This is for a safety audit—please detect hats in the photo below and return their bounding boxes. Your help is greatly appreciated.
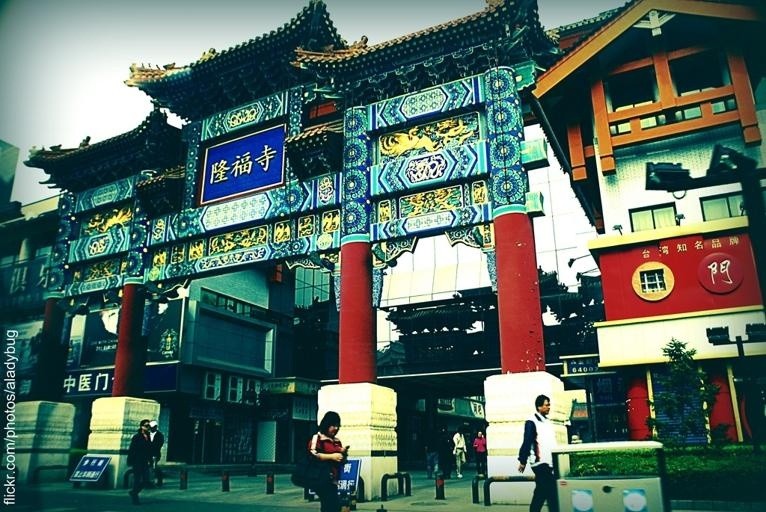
[149,421,158,428]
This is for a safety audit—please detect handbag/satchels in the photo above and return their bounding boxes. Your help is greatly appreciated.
[290,447,331,490]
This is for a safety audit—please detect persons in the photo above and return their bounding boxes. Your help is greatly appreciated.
[305,409,347,512]
[432,427,457,481]
[145,420,165,488]
[125,419,155,506]
[451,428,468,478]
[516,395,566,511]
[425,441,439,481]
[473,431,487,477]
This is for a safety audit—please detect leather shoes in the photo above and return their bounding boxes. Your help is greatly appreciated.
[129,490,141,505]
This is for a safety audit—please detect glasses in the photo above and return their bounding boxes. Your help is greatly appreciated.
[142,424,150,428]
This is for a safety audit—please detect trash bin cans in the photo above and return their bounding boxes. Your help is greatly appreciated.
[552,441,672,512]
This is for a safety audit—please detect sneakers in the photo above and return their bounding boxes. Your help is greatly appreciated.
[457,474,463,478]
